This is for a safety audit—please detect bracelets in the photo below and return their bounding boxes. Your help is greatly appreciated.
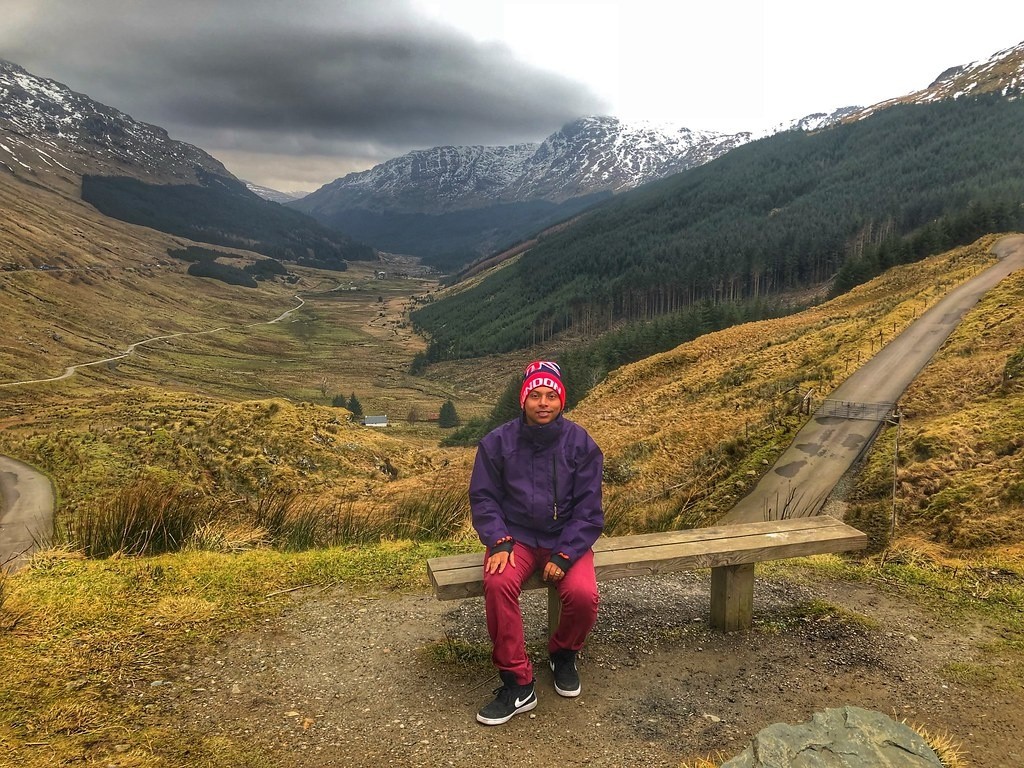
[558,552,569,559]
[494,536,513,549]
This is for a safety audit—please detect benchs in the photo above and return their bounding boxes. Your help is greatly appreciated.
[427,516,869,641]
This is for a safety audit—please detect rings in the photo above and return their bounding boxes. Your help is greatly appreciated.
[556,570,561,574]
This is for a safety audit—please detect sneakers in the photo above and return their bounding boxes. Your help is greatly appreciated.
[476,671,537,726]
[548,650,583,697]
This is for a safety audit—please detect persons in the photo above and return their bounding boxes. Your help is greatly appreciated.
[468,361,603,731]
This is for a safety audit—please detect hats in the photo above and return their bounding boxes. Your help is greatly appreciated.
[520,361,566,414]
